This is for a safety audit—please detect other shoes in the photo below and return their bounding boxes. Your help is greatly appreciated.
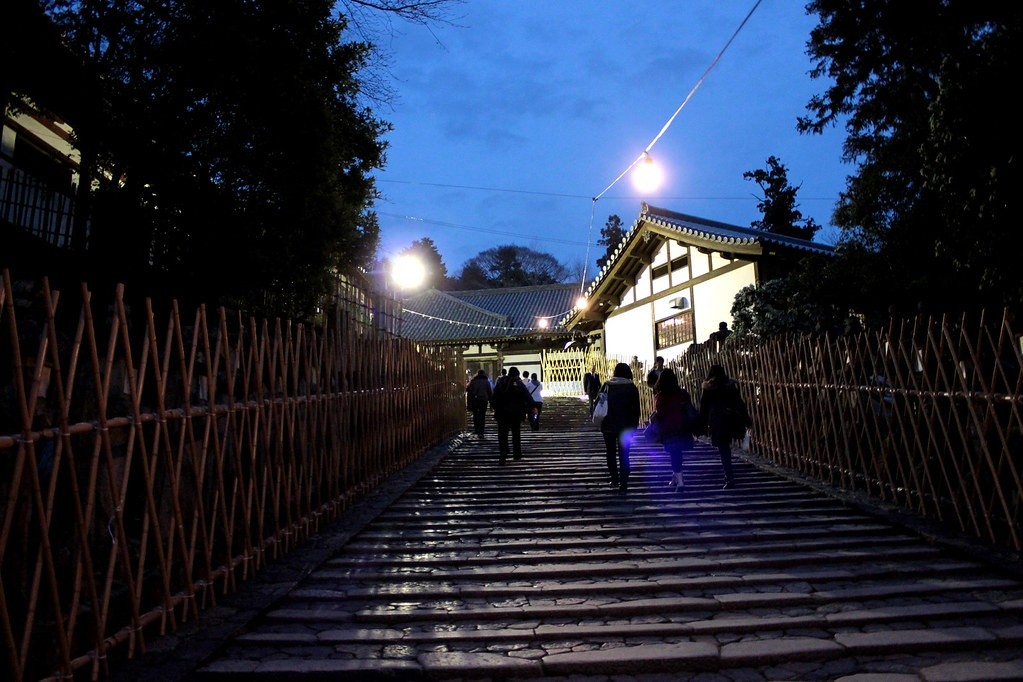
[499,450,507,466]
[609,482,619,488]
[477,433,484,439]
[723,475,735,490]
[513,453,521,461]
[618,489,626,495]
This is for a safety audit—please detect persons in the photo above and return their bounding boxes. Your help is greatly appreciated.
[644,368,699,493]
[700,365,753,490]
[710,322,733,344]
[584,365,601,417]
[485,369,507,413]
[522,371,543,431]
[466,370,494,439]
[592,363,641,495]
[491,367,538,464]
[629,356,643,381]
[647,357,665,412]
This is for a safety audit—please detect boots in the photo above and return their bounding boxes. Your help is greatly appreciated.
[669,472,685,492]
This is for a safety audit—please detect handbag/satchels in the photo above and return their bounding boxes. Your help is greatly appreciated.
[591,383,609,426]
[725,414,746,441]
